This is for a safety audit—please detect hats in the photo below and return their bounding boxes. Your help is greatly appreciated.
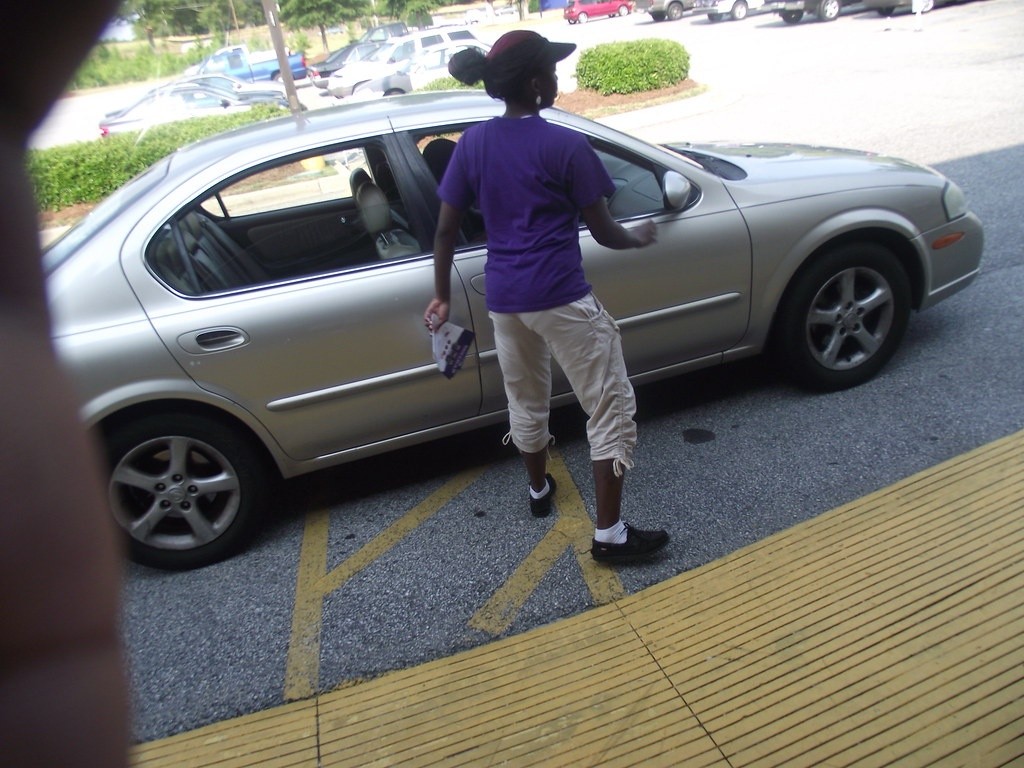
[482,30,577,84]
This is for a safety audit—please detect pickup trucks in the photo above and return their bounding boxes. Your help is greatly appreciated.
[215,45,291,62]
[196,51,307,83]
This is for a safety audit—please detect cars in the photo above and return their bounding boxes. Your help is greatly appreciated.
[352,39,492,96]
[647,0,965,23]
[99,72,290,137]
[358,22,409,39]
[564,0,633,24]
[308,40,383,98]
[31,95,987,575]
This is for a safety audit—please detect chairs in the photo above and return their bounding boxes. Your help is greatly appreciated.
[158,209,271,297]
[349,166,424,264]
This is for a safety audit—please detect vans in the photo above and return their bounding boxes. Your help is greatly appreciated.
[326,27,478,94]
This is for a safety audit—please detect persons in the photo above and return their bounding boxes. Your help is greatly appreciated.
[423,30,669,563]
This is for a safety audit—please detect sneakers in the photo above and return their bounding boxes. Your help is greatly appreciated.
[529,473,556,518]
[591,523,670,561]
